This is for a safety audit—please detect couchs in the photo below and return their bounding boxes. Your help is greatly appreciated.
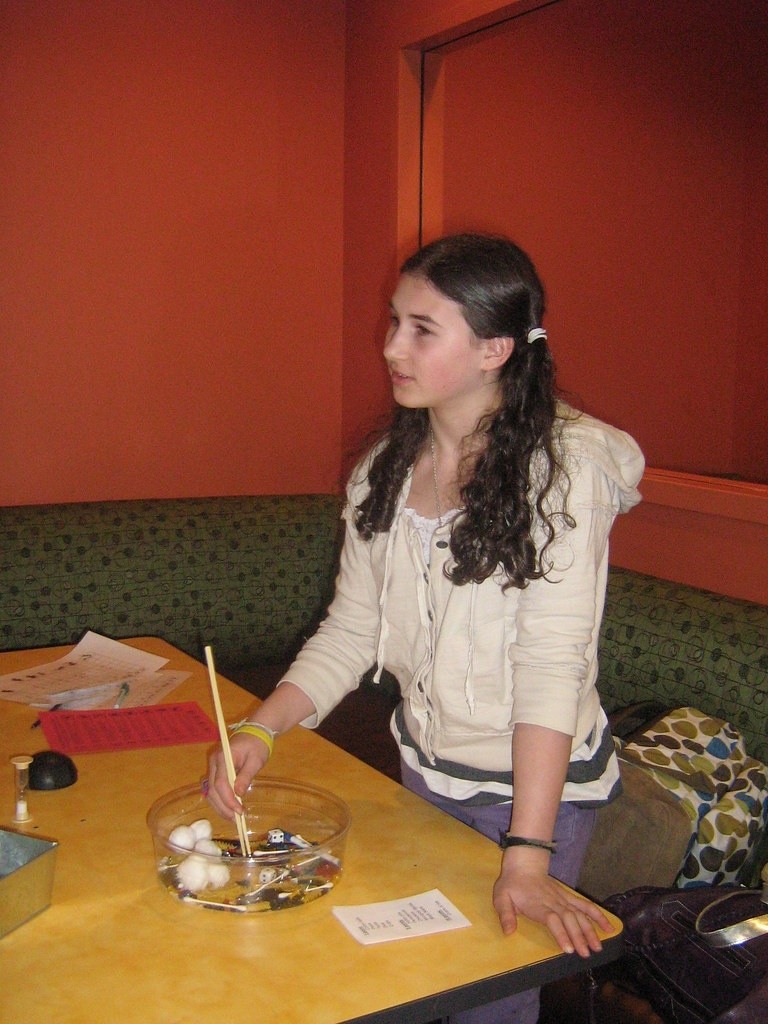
[0,495,768,1024]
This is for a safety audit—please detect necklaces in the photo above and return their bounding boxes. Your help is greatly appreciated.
[430,427,443,527]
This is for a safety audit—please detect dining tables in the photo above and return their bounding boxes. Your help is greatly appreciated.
[0,633,625,1024]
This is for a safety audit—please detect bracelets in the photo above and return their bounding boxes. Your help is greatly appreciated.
[502,836,556,852]
[227,717,274,757]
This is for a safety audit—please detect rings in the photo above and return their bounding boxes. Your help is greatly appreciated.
[202,778,210,797]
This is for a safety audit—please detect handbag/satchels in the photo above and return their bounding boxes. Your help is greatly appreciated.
[590,883,767,1024]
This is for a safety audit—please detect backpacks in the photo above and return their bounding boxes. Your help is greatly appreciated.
[575,698,768,950]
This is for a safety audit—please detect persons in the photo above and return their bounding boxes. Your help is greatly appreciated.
[204,230,645,1024]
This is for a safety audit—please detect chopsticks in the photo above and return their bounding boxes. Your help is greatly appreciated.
[205,645,252,857]
